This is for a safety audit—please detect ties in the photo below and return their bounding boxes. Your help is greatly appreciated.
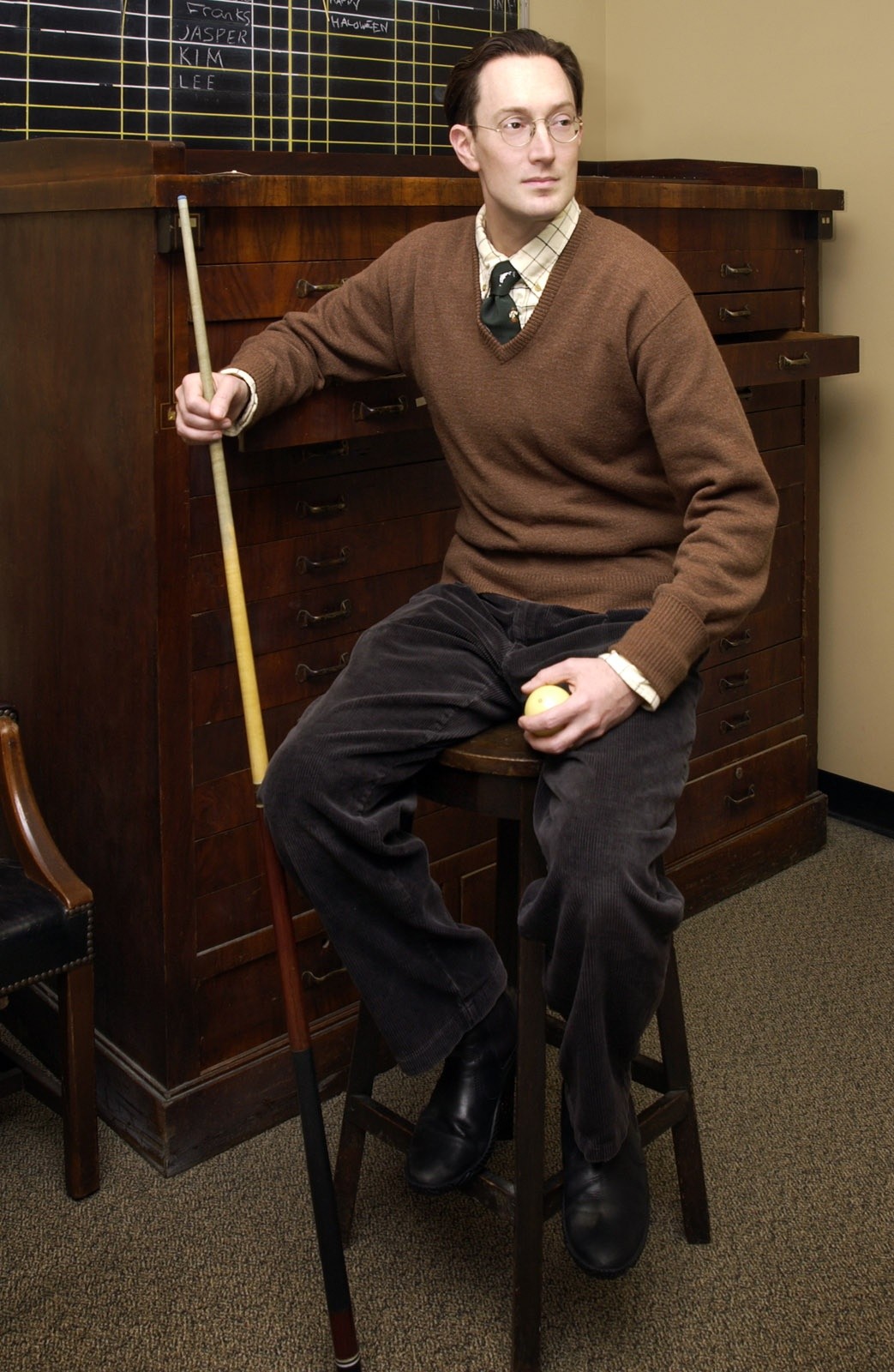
[479,259,522,347]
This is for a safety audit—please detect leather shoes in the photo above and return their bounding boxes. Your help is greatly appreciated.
[559,1085,653,1278]
[403,995,517,1196]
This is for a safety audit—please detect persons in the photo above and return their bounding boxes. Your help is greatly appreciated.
[173,28,777,1276]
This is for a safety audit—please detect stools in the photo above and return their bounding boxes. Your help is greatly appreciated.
[331,717,712,1372]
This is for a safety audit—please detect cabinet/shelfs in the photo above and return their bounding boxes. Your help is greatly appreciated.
[0,140,858,1175]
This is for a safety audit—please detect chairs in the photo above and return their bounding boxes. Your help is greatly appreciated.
[0,717,99,1199]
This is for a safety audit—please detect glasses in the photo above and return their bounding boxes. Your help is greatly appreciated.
[464,110,583,146]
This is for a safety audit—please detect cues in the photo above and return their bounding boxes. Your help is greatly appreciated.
[175,194,368,1372]
